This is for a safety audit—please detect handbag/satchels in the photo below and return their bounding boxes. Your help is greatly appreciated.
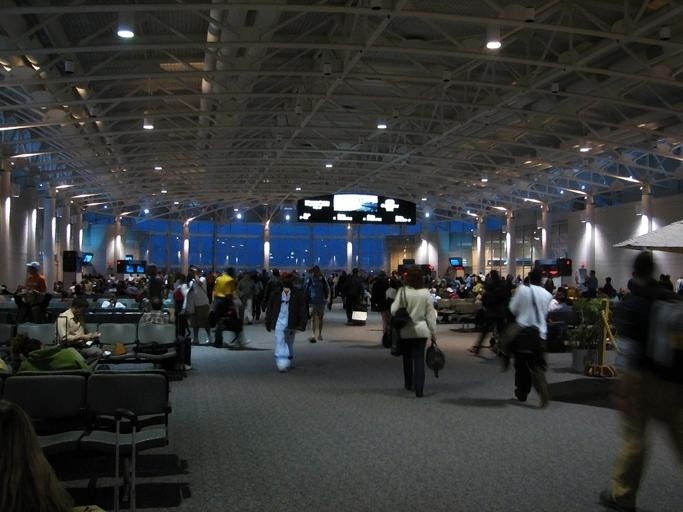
[426,340,446,374]
[391,306,413,330]
[514,326,541,358]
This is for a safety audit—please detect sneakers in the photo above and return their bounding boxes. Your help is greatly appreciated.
[467,339,497,357]
[598,487,640,512]
[401,384,424,398]
[192,316,323,373]
[517,395,549,408]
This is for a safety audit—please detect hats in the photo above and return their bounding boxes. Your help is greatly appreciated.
[26,262,41,270]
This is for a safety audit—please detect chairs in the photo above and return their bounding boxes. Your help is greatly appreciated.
[0,286,196,512]
[541,305,616,349]
[429,287,486,332]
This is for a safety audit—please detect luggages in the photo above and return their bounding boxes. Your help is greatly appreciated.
[351,299,368,326]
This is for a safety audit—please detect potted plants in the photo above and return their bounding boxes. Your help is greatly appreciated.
[570,296,610,374]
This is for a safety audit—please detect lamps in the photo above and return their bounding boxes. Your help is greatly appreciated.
[114,10,136,39]
[63,57,74,74]
[141,108,155,131]
[273,1,672,143]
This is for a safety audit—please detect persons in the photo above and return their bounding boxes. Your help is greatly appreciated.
[598,250,682,511]
[0,400,106,510]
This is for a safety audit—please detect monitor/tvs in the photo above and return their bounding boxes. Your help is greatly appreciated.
[124,264,136,274]
[449,257,462,267]
[125,255,133,260]
[82,253,94,264]
[136,264,145,273]
